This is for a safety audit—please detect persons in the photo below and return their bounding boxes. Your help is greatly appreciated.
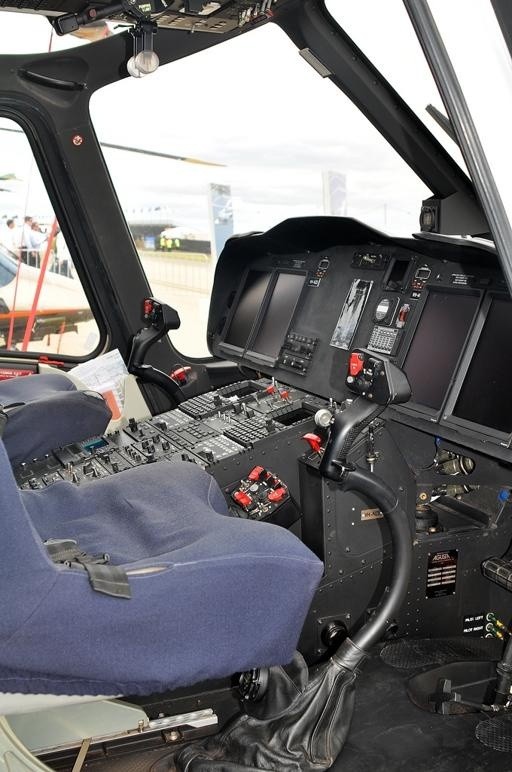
[5,218,17,251]
[29,222,51,268]
[16,216,38,266]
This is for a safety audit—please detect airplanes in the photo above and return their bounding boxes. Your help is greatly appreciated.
[0,242,95,347]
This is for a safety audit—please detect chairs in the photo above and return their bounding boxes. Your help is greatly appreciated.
[1,371,112,464]
[1,441,323,717]
